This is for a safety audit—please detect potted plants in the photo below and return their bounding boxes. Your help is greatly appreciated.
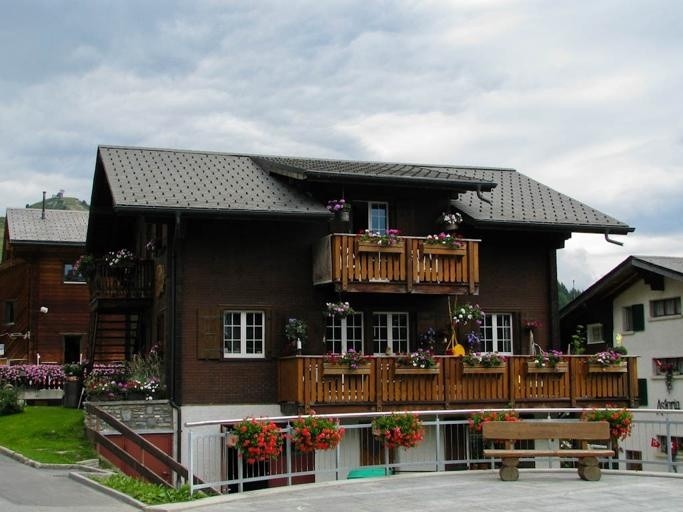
[275,312,311,353]
[63,362,83,382]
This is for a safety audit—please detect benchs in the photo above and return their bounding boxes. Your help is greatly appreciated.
[480,418,617,481]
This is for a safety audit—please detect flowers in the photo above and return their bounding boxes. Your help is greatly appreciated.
[324,198,350,213]
[339,347,366,369]
[529,348,564,369]
[370,406,425,452]
[359,227,465,251]
[86,359,163,403]
[436,210,463,226]
[226,407,348,465]
[0,362,65,385]
[404,299,508,370]
[468,406,522,445]
[580,403,635,442]
[591,349,624,372]
[323,301,357,318]
[523,318,545,334]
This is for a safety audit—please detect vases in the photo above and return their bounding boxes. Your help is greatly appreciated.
[338,211,349,221]
[445,224,459,232]
